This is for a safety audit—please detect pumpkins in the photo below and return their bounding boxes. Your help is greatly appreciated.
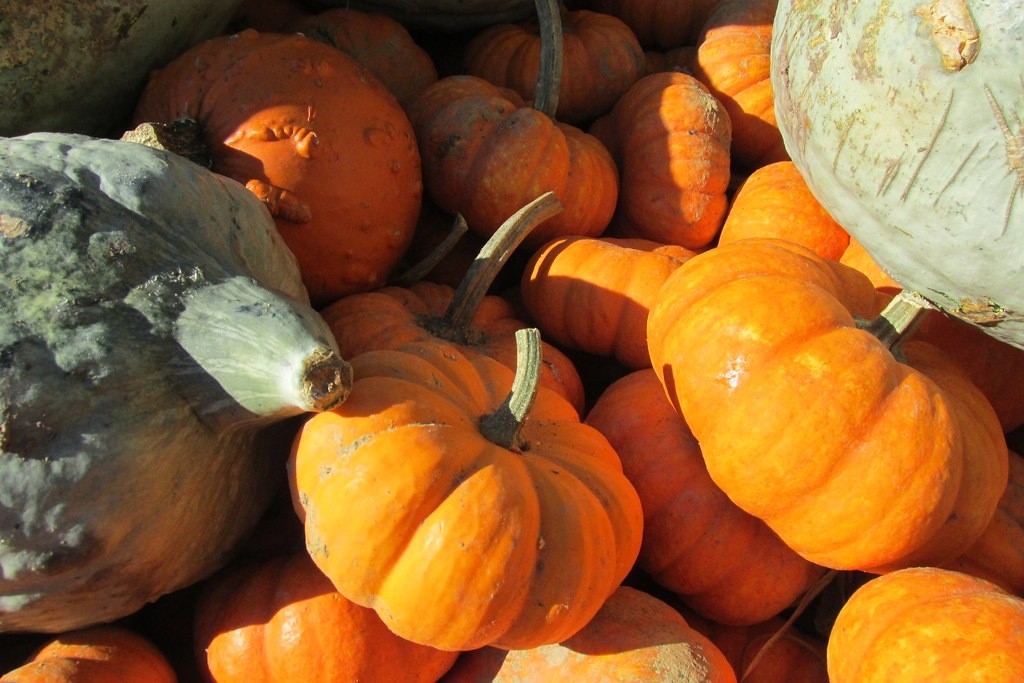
[0,0,1024,682]
